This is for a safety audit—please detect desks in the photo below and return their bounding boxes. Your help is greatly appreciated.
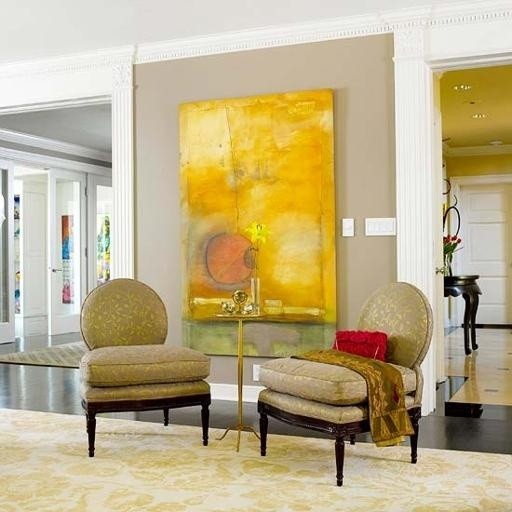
[442,274,482,355]
[214,312,266,453]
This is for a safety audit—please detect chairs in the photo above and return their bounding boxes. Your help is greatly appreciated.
[79,277,211,457]
[258,282,434,486]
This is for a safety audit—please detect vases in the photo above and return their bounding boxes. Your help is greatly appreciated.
[443,260,450,276]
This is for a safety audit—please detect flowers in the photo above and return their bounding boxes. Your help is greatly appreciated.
[442,234,464,274]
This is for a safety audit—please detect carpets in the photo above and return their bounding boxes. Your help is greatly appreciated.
[1,339,88,369]
[1,406,512,510]
